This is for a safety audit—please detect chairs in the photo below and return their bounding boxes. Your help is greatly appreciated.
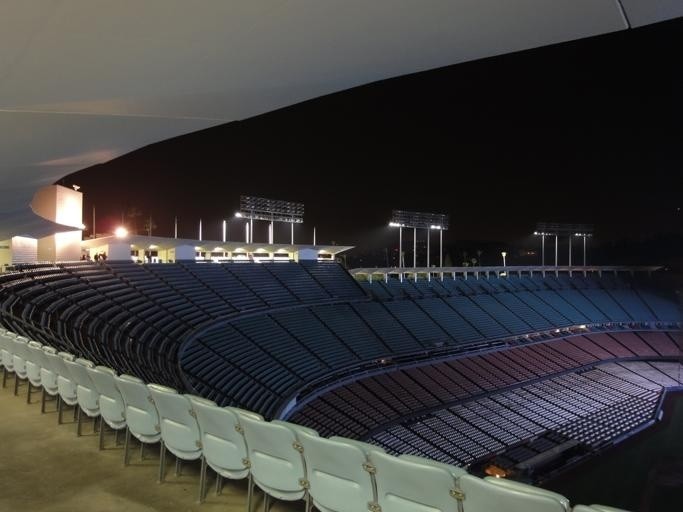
[0,327,631,512]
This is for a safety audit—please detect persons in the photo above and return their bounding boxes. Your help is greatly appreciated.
[82,252,108,265]
